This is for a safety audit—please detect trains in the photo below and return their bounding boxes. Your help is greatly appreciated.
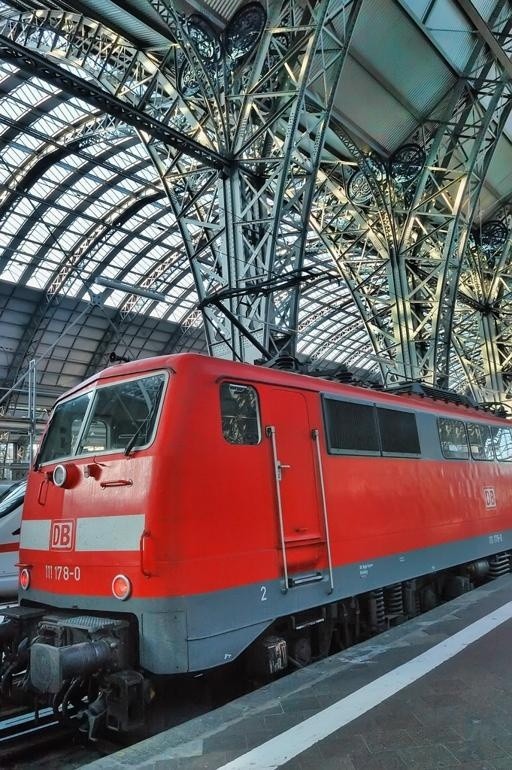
[1,472,33,604]
[0,350,512,754]
